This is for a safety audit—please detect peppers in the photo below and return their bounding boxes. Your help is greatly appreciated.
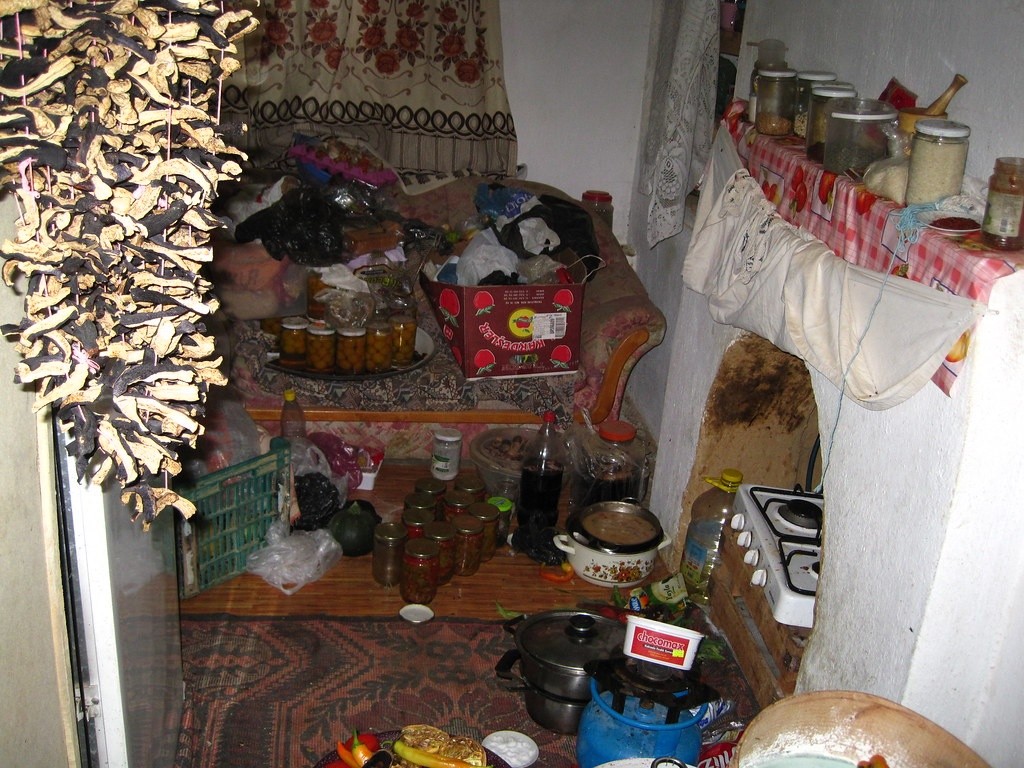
[378,740,494,768]
[539,560,574,583]
[495,600,527,618]
[324,728,401,768]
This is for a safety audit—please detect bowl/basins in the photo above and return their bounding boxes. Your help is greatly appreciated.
[622,616,705,670]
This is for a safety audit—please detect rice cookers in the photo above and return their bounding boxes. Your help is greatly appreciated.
[553,497,672,587]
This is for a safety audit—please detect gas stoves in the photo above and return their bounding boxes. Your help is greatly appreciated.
[731,483,824,628]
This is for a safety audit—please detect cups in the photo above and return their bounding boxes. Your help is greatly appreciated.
[758,40,784,70]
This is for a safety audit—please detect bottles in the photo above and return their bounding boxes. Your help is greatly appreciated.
[679,468,743,606]
[574,419,649,505]
[981,157,1024,251]
[280,270,417,374]
[582,189,613,233]
[373,475,518,606]
[511,410,565,552]
[431,428,462,480]
[750,61,970,207]
[280,389,307,438]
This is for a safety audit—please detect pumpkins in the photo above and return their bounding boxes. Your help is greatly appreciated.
[328,505,378,557]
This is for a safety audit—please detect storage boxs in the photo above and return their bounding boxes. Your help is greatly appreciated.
[418,243,588,381]
[175,436,294,597]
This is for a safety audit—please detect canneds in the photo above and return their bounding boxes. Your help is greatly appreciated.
[981,156,1024,251]
[431,428,462,480]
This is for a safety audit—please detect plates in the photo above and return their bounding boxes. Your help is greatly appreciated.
[312,731,512,768]
[916,210,983,236]
[265,326,435,381]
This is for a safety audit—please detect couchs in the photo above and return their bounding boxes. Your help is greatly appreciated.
[205,166,666,461]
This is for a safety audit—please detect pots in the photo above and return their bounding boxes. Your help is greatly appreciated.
[493,612,628,736]
